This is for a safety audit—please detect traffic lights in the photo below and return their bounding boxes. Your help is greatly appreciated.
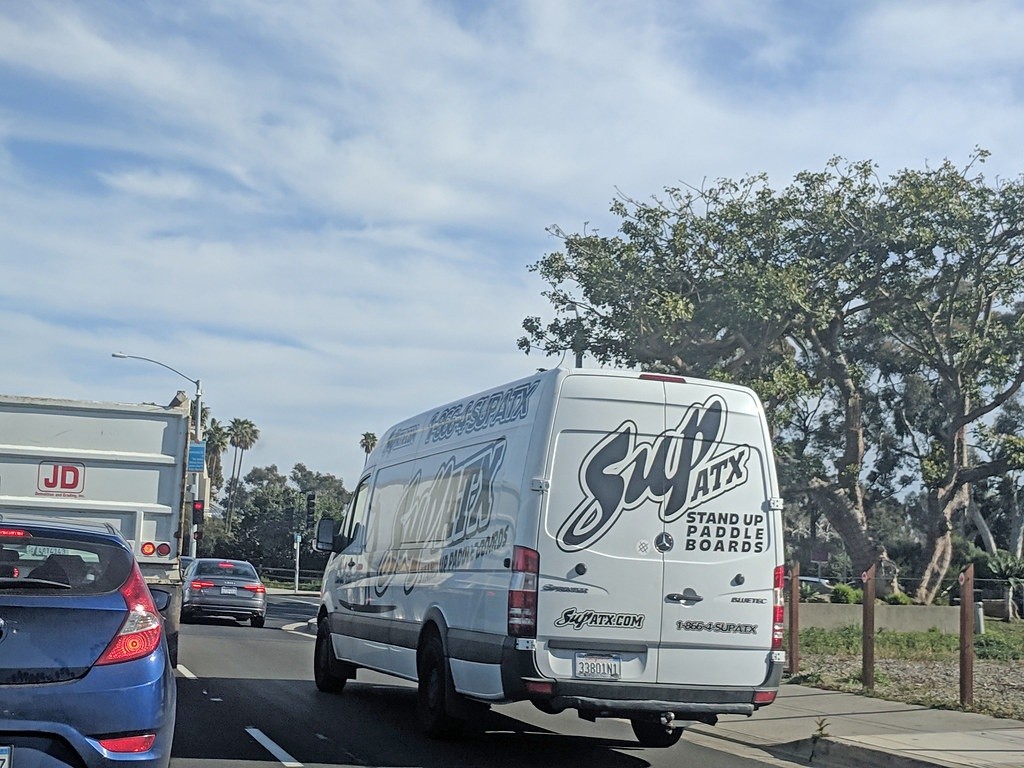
[191,499,204,525]
[305,494,315,528]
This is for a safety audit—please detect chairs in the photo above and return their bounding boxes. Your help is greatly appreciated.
[0,550,96,586]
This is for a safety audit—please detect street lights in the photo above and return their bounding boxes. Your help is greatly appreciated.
[110,351,206,559]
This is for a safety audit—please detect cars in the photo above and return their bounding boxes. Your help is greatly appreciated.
[0,515,177,768]
[175,554,193,581]
[783,574,829,604]
[180,559,267,628]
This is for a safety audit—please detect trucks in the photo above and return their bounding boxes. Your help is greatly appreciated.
[0,388,199,672]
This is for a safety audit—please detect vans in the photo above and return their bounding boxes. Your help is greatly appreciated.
[308,368,788,748]
